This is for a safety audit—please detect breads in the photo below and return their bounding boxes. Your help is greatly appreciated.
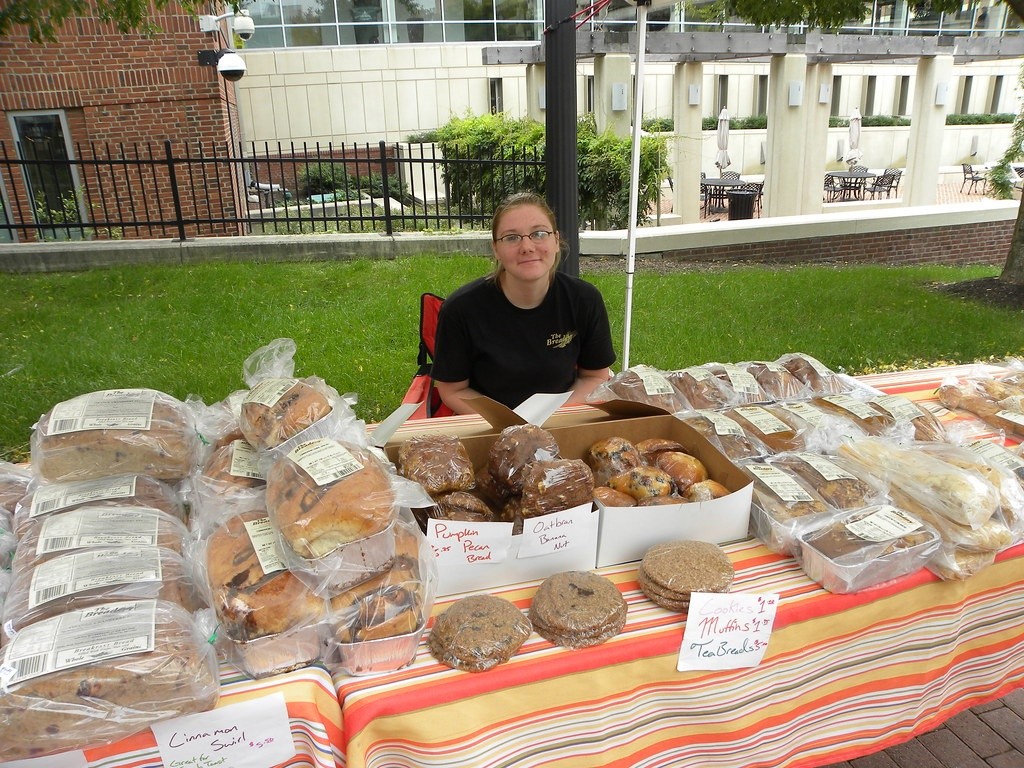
[399,423,735,534]
[0,380,423,766]
[608,355,1024,582]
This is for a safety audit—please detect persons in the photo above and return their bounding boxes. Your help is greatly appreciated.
[428,191,618,416]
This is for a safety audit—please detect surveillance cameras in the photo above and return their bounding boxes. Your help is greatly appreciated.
[218,53,247,81]
[235,17,255,40]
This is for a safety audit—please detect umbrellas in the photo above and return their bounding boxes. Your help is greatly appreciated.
[711,105,732,213]
[844,107,864,201]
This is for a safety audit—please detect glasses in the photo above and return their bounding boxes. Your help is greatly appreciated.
[496,230,556,246]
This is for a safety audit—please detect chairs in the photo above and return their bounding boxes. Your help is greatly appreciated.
[960,163,986,196]
[403,291,455,420]
[668,171,765,219]
[824,165,903,202]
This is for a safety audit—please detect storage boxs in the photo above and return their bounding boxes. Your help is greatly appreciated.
[372,395,601,599]
[545,399,757,568]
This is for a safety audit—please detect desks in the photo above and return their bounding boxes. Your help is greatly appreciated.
[829,172,877,201]
[701,178,746,219]
[0,362,1024,768]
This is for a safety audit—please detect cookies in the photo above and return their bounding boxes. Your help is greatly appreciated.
[525,571,628,649]
[637,542,734,615]
[426,595,532,673]
